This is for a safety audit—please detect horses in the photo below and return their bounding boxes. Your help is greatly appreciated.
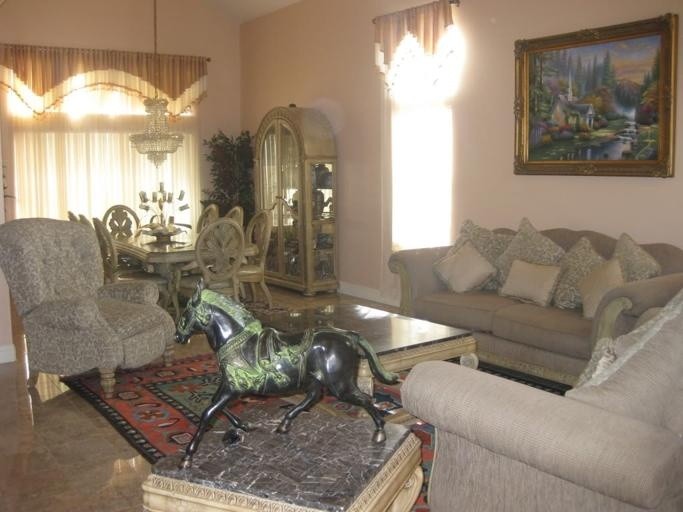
[171,276,403,471]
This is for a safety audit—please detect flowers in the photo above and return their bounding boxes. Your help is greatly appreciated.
[139,183,190,224]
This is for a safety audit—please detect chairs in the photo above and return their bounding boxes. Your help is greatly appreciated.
[0,218,176,399]
[400,307,683,512]
[68,203,273,321]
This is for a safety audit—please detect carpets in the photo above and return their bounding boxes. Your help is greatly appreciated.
[58,353,573,512]
[167,294,292,321]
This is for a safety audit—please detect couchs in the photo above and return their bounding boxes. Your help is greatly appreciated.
[388,228,683,386]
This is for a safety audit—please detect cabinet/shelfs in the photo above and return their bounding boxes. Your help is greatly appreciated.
[255,106,339,296]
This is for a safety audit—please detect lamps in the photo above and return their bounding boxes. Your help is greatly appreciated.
[129,0,184,168]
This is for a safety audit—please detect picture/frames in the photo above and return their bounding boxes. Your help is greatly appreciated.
[514,13,679,177]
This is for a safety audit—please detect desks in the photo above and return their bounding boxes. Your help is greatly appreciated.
[141,403,424,512]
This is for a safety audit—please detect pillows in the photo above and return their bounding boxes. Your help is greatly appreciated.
[577,289,682,387]
[433,217,660,319]
